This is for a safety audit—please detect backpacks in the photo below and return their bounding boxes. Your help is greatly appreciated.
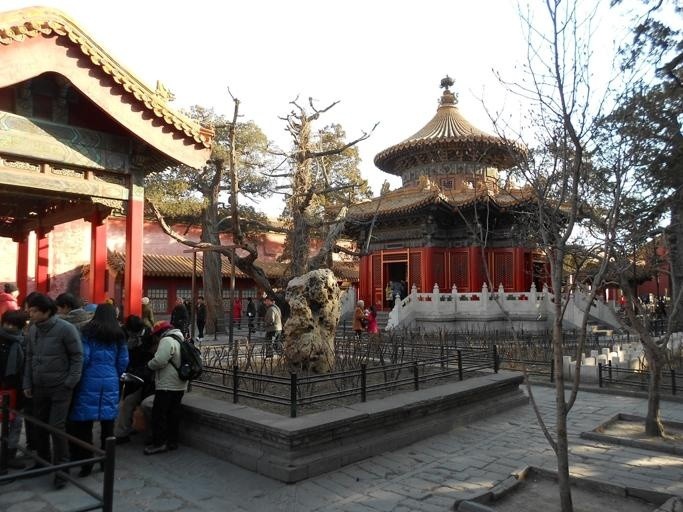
[162,326,203,383]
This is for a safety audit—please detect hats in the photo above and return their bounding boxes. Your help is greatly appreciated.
[147,319,174,336]
[125,315,144,332]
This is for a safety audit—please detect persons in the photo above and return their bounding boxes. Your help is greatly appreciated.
[352,300,367,341]
[385,279,406,309]
[232,292,285,357]
[365,306,377,334]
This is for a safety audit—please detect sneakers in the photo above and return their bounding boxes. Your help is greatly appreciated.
[142,439,178,456]
[16,449,37,458]
[75,465,93,479]
[51,464,72,490]
[116,436,130,444]
[6,461,25,469]
[24,460,54,479]
[129,428,137,436]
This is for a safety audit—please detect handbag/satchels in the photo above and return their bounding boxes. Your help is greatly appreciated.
[361,318,369,329]
